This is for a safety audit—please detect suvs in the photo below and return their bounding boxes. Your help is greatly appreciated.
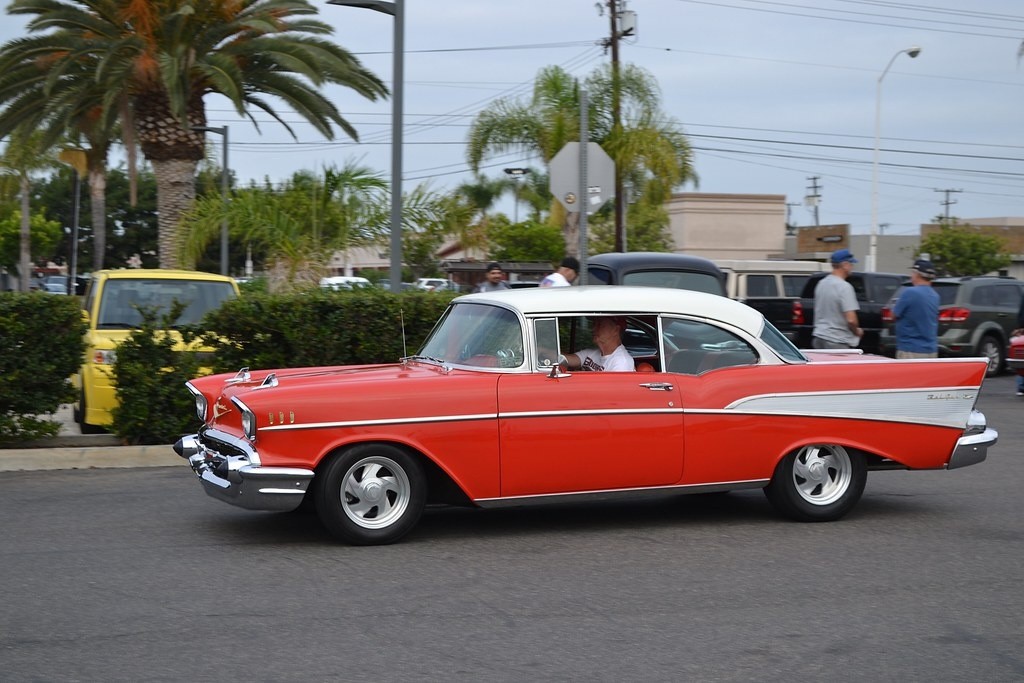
[876,275,1024,377]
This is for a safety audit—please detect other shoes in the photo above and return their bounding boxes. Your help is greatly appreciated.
[1016,388,1024,395]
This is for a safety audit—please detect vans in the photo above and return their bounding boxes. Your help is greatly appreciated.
[792,270,914,350]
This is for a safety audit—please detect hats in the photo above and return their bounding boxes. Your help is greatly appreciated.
[560,256,580,273]
[831,249,858,264]
[486,263,501,271]
[908,259,936,275]
[586,316,628,330]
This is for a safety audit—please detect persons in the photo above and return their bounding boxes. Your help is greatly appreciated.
[810,248,864,349]
[893,260,941,358]
[474,261,511,292]
[1017,376,1023,394]
[539,257,581,286]
[527,315,636,371]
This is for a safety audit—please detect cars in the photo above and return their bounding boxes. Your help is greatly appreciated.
[1003,333,1024,379]
[312,250,835,344]
[60,268,251,435]
[175,282,996,542]
[8,269,94,298]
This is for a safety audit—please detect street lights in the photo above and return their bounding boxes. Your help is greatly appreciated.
[868,44,924,270]
[325,0,408,292]
